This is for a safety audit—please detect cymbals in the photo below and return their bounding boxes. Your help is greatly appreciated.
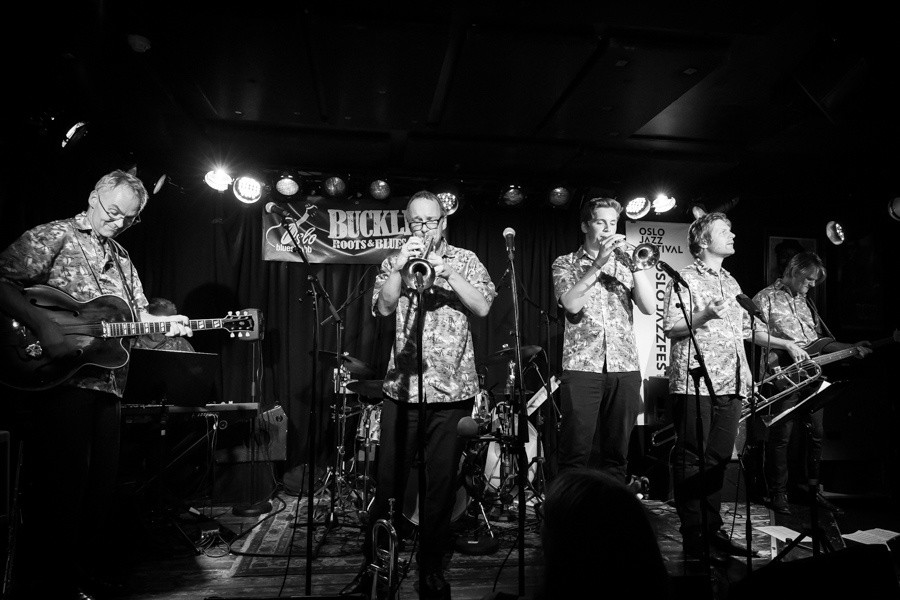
[345,380,384,399]
[309,348,379,375]
[492,387,535,396]
[488,344,543,361]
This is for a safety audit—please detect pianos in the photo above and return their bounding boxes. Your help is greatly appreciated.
[117,400,260,555]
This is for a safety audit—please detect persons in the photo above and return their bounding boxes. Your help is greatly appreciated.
[339,189,497,600]
[0,170,197,600]
[552,198,656,488]
[751,248,872,516]
[663,212,755,557]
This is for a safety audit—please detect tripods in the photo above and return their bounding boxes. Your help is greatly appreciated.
[760,378,852,562]
[286,360,364,522]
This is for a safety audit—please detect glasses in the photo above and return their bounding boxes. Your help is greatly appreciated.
[408,214,445,233]
[98,195,140,226]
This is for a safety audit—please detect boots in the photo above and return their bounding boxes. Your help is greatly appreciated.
[773,463,800,514]
[804,454,837,511]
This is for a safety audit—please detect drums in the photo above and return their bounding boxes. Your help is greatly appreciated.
[401,451,476,528]
[354,404,382,446]
[470,388,491,427]
[479,417,545,500]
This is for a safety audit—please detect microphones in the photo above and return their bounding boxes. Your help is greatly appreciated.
[265,202,293,218]
[736,293,769,325]
[660,262,689,289]
[376,266,381,275]
[495,270,510,293]
[520,354,536,375]
[502,227,517,260]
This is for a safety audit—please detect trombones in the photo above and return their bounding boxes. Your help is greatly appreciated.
[650,356,823,447]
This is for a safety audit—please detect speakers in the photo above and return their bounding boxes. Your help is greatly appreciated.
[231,406,289,462]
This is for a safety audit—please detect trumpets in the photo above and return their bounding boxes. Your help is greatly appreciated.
[602,236,660,270]
[367,497,398,599]
[400,236,436,291]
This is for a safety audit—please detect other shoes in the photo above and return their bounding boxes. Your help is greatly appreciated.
[341,571,371,595]
[683,536,734,558]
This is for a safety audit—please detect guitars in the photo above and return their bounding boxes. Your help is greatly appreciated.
[0,284,256,394]
[763,328,900,400]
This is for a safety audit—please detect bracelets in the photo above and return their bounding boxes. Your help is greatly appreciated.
[592,261,601,269]
